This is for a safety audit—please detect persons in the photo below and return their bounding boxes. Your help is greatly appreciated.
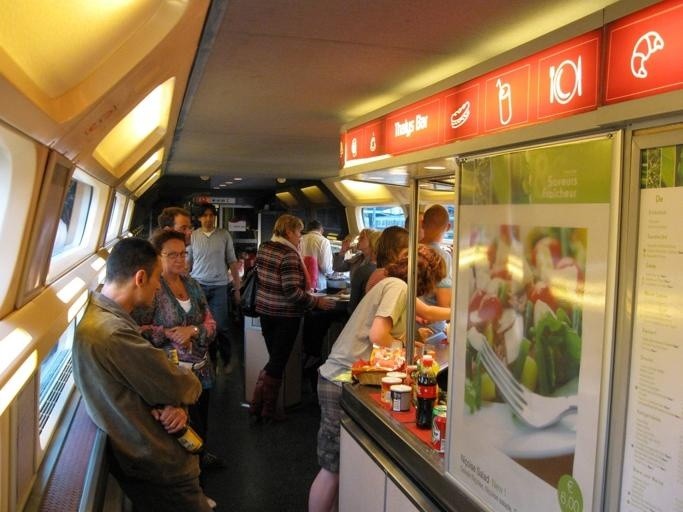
[72,237,214,512]
[307,243,451,512]
[132,203,241,487]
[297,204,481,392]
[248,213,337,422]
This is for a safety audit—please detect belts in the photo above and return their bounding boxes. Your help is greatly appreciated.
[179,358,208,370]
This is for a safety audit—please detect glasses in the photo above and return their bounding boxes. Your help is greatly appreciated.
[162,250,188,258]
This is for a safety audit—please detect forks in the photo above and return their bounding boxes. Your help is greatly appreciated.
[478,336,577,430]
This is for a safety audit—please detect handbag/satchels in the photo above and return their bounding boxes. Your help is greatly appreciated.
[240,271,259,317]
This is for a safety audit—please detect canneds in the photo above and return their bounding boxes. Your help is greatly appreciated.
[380,371,411,412]
[431,404,447,454]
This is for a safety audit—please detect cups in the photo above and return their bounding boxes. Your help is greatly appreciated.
[343,241,358,248]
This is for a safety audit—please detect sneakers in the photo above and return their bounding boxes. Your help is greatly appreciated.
[216,362,232,376]
[206,496,218,510]
[205,452,226,468]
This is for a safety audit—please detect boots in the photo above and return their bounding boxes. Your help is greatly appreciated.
[248,368,282,417]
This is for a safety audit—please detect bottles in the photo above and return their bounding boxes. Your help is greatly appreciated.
[155,406,206,455]
[169,349,179,366]
[415,357,437,429]
